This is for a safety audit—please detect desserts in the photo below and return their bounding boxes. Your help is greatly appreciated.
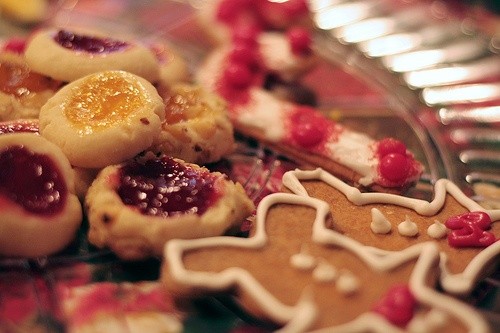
[0,25,500,333]
